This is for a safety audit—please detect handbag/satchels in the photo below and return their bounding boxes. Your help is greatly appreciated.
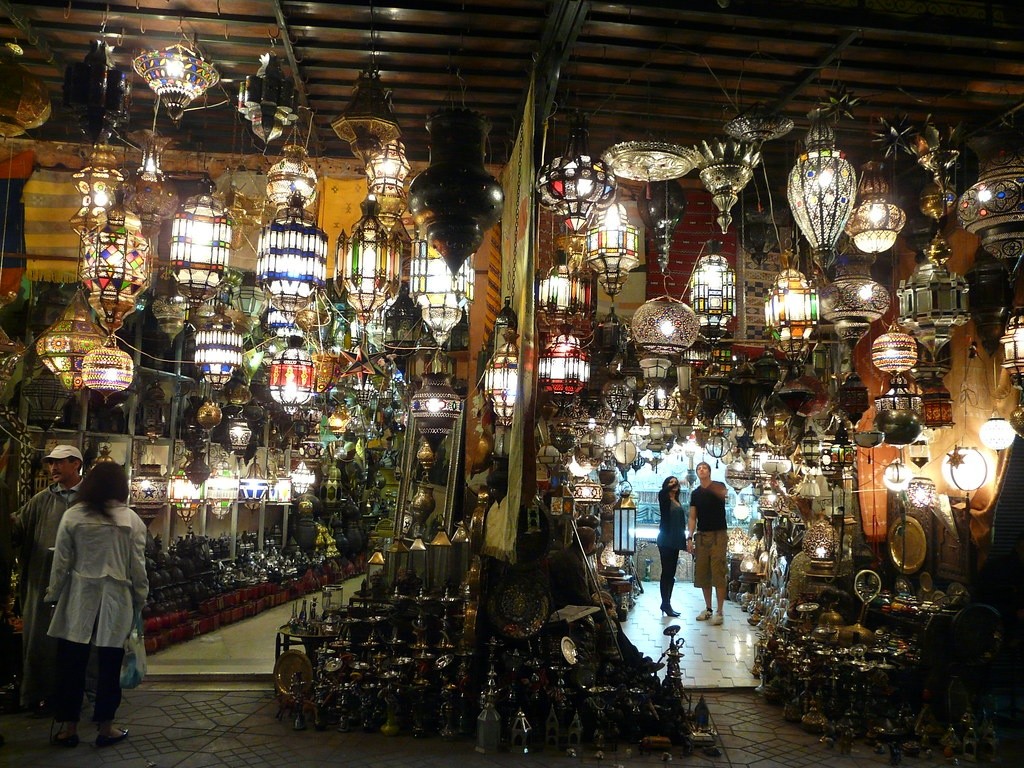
[118,607,146,688]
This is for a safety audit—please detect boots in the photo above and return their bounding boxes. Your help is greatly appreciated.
[660,577,681,617]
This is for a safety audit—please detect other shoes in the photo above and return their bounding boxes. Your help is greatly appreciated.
[696,608,712,620]
[713,615,723,625]
[53,729,79,747]
[96,726,129,746]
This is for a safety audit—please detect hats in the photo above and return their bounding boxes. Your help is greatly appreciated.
[43,445,83,462]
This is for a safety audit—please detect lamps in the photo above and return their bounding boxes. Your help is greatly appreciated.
[1,1,1024,613]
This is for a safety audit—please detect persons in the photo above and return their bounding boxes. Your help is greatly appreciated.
[657,475,687,617]
[678,462,730,626]
[0,444,86,717]
[43,461,150,749]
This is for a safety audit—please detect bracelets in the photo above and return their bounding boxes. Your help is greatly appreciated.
[687,537,692,541]
